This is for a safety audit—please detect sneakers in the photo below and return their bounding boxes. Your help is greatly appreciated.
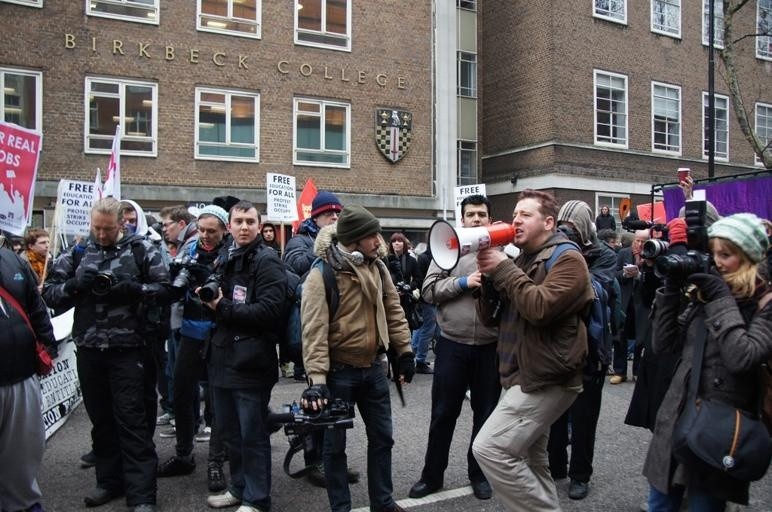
[85,487,124,506]
[207,465,263,512]
[610,375,626,384]
[157,456,195,476]
[80,451,96,466]
[306,464,358,487]
[156,413,211,442]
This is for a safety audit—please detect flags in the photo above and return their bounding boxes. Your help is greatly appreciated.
[104,124,122,202]
[92,167,103,207]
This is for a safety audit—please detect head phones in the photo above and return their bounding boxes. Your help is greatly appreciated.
[336,241,363,266]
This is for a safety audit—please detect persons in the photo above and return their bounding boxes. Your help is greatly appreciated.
[1,174,770,511]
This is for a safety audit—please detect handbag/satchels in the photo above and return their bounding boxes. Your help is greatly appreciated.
[410,303,424,330]
[672,397,772,481]
[35,341,53,375]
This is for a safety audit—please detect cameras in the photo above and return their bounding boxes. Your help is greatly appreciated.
[641,239,669,258]
[653,200,712,279]
[197,274,230,301]
[88,269,116,296]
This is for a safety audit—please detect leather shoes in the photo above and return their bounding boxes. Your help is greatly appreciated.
[471,480,492,499]
[569,480,587,499]
[409,482,442,498]
[416,362,434,374]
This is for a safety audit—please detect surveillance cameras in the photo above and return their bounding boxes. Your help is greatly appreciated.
[509,175,517,184]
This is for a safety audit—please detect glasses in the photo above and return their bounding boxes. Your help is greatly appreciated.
[322,211,341,216]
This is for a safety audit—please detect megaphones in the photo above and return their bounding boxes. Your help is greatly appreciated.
[429,214,515,273]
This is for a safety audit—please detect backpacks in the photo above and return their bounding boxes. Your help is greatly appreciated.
[251,246,339,362]
[547,243,612,374]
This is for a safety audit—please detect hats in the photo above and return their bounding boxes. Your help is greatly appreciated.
[311,190,380,246]
[707,212,772,263]
[198,205,229,226]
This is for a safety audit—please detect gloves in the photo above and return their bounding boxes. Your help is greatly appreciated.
[109,273,138,298]
[688,266,730,301]
[65,268,97,295]
